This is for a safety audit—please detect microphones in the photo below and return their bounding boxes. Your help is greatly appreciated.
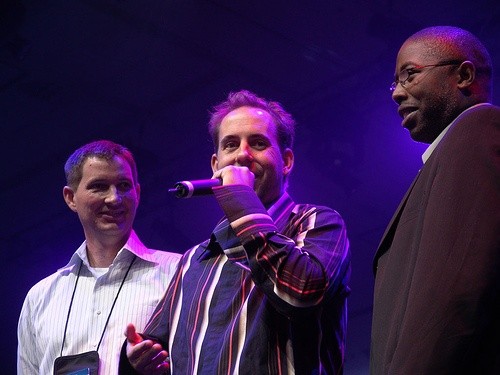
[168,178,222,198]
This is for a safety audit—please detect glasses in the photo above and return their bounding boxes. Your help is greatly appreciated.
[391,62,461,90]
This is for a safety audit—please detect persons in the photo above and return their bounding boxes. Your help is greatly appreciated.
[16,141,182,375]
[118,91,350,375]
[371,26,500,375]
